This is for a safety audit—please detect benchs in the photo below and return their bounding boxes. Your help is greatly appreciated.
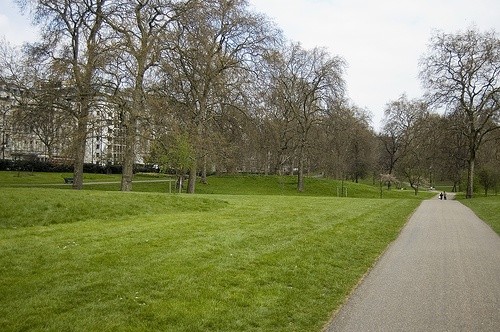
[64,178,73,184]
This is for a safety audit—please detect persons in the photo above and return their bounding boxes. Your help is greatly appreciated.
[439,192,444,200]
[442,190,447,200]
